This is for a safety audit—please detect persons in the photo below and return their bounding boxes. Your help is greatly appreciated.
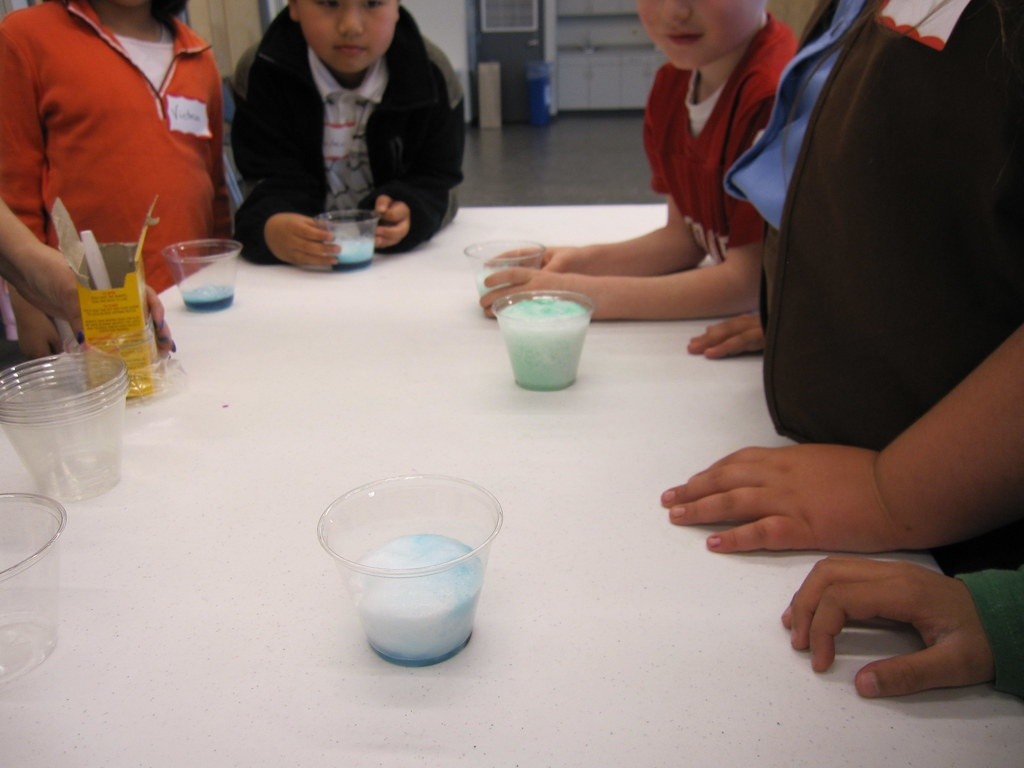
[231,0,466,276]
[0,0,236,363]
[478,0,1024,702]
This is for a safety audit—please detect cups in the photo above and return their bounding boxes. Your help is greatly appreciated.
[0,352,131,685]
[162,238,244,312]
[318,474,505,668]
[492,288,597,391]
[315,209,381,271]
[463,237,547,317]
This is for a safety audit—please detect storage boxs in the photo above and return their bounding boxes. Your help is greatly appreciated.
[50,194,165,398]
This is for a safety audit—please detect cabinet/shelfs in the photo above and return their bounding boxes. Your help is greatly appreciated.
[542,0,666,124]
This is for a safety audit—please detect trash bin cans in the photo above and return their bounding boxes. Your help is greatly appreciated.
[524,61,552,126]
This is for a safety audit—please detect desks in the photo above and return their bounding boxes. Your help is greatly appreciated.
[0,205,1024,768]
